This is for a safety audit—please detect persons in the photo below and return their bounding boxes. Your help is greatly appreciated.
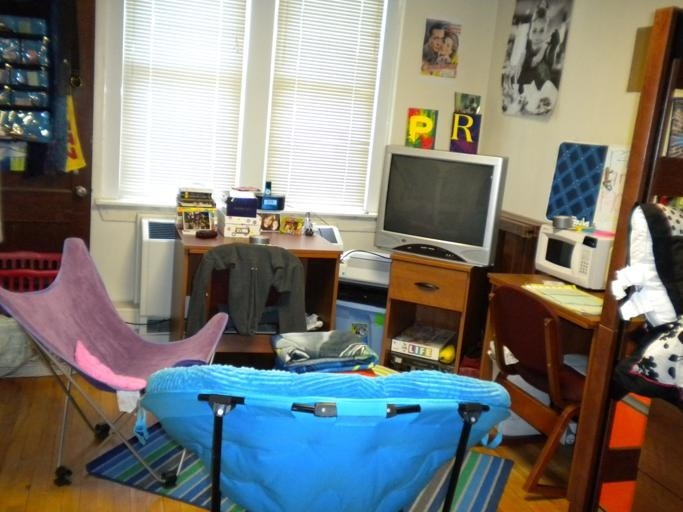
[500,0,573,115]
[436,32,459,65]
[422,21,448,65]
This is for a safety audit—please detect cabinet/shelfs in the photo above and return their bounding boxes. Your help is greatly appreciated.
[565,5,681,511]
[380,249,493,374]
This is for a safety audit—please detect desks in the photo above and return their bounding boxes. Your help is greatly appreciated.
[165,228,341,354]
[472,267,608,389]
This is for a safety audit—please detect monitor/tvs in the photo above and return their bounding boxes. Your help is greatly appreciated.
[374,144,510,268]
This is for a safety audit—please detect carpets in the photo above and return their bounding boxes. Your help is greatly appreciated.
[73,414,511,512]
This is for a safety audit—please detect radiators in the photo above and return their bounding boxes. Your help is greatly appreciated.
[134,215,192,322]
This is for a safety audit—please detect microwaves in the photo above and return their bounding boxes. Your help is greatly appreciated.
[533,223,615,291]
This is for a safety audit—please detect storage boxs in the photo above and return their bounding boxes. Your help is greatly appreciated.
[220,192,257,218]
[216,208,261,239]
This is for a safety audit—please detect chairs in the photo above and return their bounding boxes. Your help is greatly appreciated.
[477,280,586,490]
[126,364,509,512]
[191,243,308,355]
[0,231,229,492]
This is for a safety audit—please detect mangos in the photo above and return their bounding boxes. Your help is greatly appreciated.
[440,344,455,363]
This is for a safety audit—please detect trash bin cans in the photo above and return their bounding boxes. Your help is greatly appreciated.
[488,340,550,437]
